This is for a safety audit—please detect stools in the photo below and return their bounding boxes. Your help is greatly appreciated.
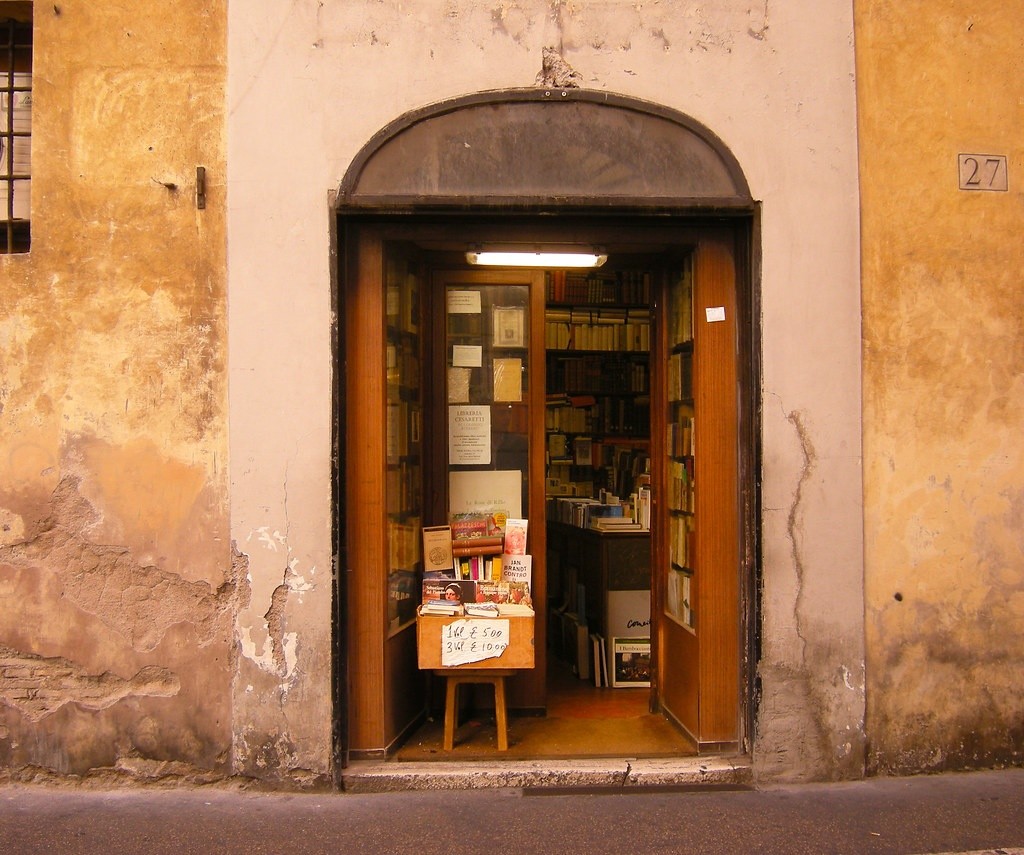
[433,671,518,751]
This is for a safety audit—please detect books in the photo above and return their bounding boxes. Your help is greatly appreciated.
[666,271,695,630]
[447,267,652,532]
[386,254,425,633]
[547,589,650,688]
[420,515,535,618]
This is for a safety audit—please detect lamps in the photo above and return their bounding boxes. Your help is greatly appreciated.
[466,253,609,268]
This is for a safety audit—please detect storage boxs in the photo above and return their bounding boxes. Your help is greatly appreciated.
[415,603,535,670]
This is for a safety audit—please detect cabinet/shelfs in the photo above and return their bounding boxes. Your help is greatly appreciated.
[546,302,653,690]
